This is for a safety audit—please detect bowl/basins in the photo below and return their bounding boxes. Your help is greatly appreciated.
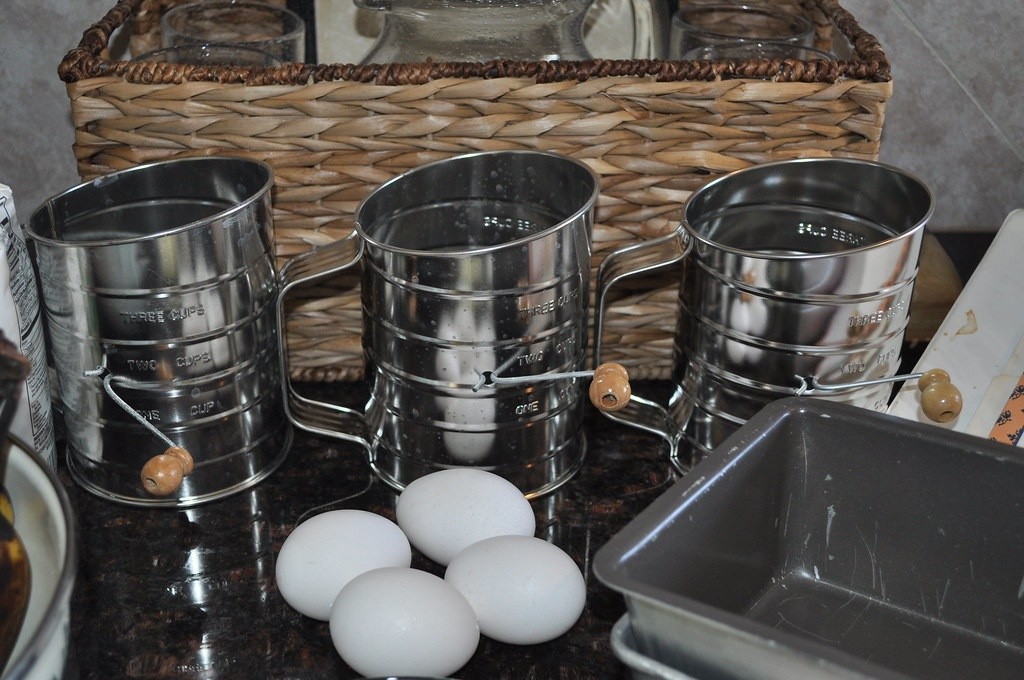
[0,430,81,680]
[588,391,1023,680]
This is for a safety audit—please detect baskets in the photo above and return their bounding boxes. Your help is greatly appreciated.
[57,1,890,382]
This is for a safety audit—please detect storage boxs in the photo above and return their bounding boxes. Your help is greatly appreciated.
[593,398,1024,680]
[58,1,895,380]
[272,149,599,504]
[599,158,935,489]
[24,156,288,510]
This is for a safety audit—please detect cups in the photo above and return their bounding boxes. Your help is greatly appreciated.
[26,148,294,507]
[693,41,837,63]
[128,42,283,77]
[587,153,962,486]
[267,148,634,503]
[159,0,306,69]
[665,7,817,64]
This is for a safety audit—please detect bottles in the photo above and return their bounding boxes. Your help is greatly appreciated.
[348,0,653,62]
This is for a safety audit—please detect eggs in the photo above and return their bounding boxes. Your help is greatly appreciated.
[276,467,587,680]
